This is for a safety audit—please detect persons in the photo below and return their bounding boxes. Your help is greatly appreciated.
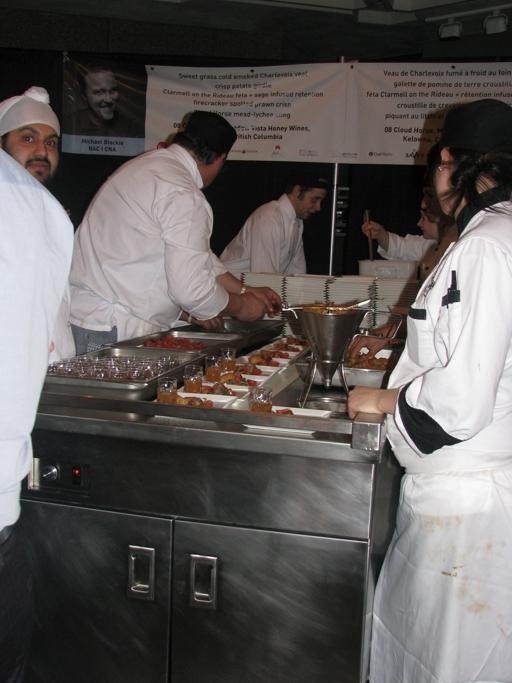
[64,60,145,136]
[70,109,285,350]
[0,79,64,185]
[345,98,511,682]
[218,170,330,276]
[179,309,228,330]
[0,146,79,683]
[358,186,454,261]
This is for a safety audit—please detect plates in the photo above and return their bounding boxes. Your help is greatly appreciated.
[241,268,421,334]
[148,337,394,439]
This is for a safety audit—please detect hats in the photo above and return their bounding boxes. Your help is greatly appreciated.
[185,110,237,154]
[427,98,512,164]
[0,85,61,137]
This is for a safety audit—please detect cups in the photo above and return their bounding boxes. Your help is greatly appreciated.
[48,354,178,379]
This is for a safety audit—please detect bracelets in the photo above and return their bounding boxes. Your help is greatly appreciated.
[188,314,193,322]
[240,285,249,294]
